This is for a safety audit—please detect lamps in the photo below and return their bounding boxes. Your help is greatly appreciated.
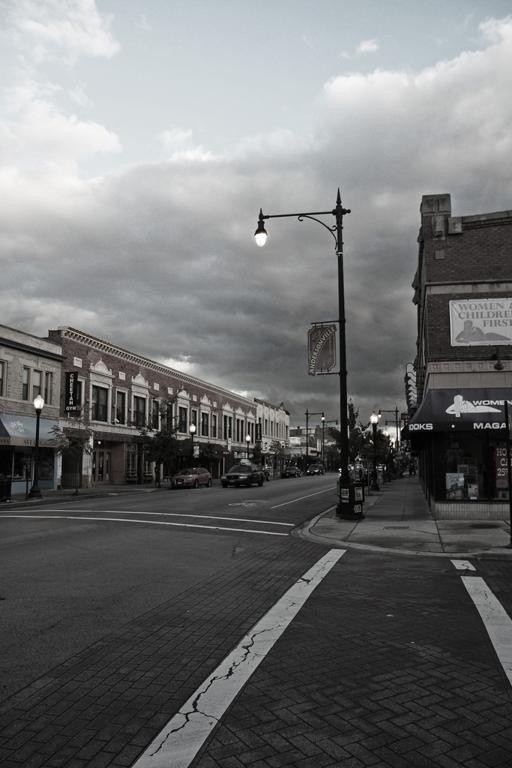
[491,352,505,370]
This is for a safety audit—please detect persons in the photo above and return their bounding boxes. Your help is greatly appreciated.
[359,468,362,478]
[154,463,160,488]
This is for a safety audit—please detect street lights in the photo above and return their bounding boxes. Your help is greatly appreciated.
[246,434,252,458]
[253,187,355,516]
[321,416,326,465]
[189,423,197,468]
[369,406,402,491]
[29,393,45,497]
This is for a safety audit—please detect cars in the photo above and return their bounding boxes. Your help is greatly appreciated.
[222,459,327,489]
[171,468,213,489]
